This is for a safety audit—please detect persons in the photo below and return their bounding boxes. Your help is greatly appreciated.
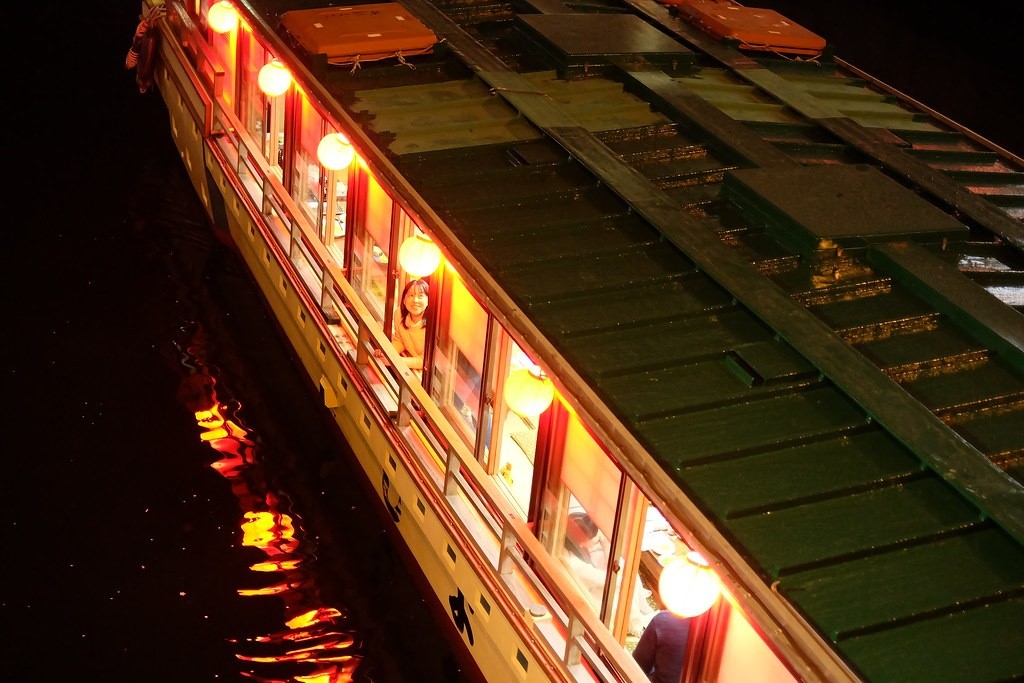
[632,609,691,683]
[373,279,429,370]
